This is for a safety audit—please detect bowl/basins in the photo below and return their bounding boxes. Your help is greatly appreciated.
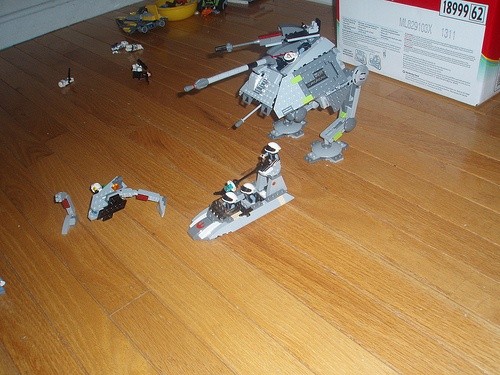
[153,0,198,21]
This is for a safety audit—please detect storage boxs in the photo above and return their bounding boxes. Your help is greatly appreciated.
[335,0,500,107]
